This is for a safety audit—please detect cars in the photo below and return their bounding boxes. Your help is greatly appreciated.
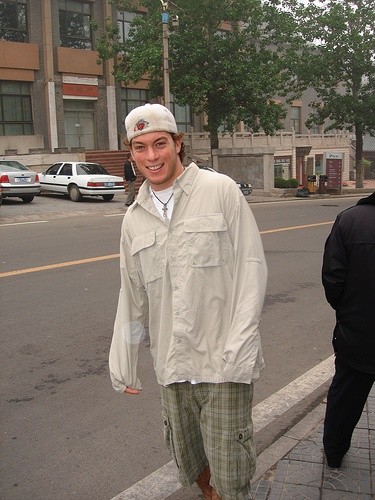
[198,165,252,196]
[0,160,42,203]
[32,161,125,202]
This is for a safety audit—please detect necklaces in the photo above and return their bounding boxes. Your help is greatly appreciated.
[152,190,174,218]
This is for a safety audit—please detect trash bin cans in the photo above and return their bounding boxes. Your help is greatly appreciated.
[319,175,328,194]
[307,175,316,193]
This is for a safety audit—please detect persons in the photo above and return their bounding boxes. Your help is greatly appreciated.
[124,153,137,206]
[322,192,375,468]
[109,104,267,500]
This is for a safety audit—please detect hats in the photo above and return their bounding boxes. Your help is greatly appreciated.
[124,102,178,145]
